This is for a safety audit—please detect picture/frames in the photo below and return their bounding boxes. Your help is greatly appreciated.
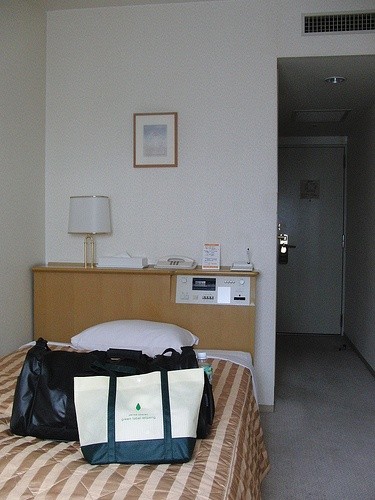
[133,112,178,167]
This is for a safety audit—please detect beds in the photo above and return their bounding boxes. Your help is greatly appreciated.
[0,340,271,499]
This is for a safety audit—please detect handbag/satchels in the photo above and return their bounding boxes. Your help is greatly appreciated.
[9,337,215,442]
[73,368,204,465]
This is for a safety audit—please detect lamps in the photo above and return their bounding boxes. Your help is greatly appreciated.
[68,196,112,268]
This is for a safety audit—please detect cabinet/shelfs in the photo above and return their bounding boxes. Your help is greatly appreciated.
[32,265,259,364]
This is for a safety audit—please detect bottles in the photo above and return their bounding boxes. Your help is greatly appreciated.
[197,352,213,384]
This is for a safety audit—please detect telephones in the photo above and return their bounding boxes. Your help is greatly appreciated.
[153,255,198,269]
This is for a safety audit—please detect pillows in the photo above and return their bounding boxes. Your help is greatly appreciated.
[71,319,199,357]
